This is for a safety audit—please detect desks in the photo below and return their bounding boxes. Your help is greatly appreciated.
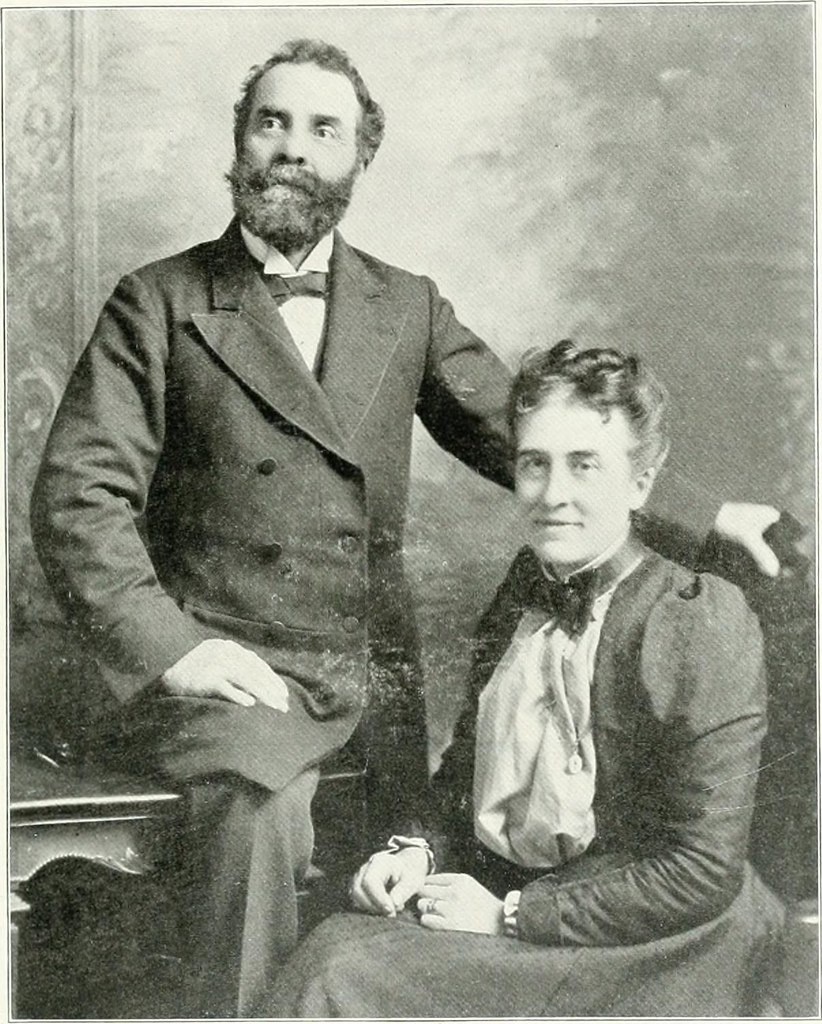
[10,731,367,923]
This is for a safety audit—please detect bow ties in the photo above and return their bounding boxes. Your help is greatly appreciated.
[525,526,643,636]
[251,254,331,307]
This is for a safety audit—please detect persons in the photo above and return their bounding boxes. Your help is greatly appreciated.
[246,338,819,1017]
[29,38,803,1018]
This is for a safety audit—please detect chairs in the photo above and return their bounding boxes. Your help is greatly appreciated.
[636,510,820,1016]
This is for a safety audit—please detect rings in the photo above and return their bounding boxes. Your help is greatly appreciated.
[427,899,436,914]
